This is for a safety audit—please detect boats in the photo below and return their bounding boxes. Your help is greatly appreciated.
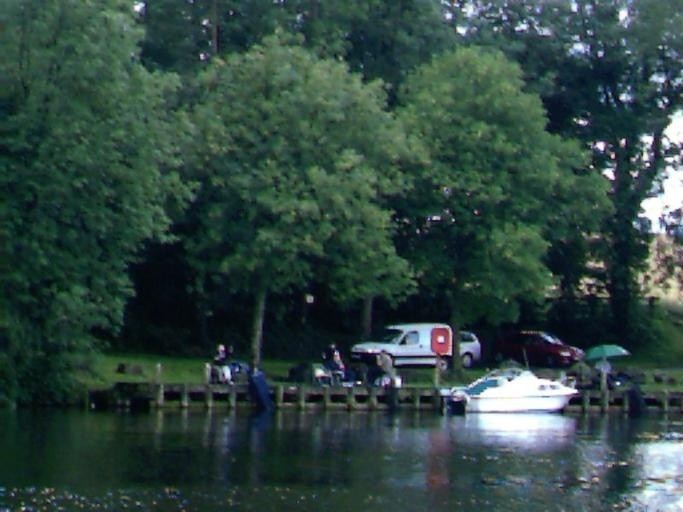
[435,364,582,415]
[446,411,577,455]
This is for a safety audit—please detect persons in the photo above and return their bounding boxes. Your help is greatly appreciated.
[595,358,613,379]
[211,341,397,390]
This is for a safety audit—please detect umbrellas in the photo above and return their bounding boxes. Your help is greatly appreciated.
[582,344,631,358]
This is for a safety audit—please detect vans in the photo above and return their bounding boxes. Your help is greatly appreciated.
[348,320,453,372]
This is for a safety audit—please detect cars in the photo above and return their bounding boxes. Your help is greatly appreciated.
[506,331,584,367]
[449,330,481,367]
